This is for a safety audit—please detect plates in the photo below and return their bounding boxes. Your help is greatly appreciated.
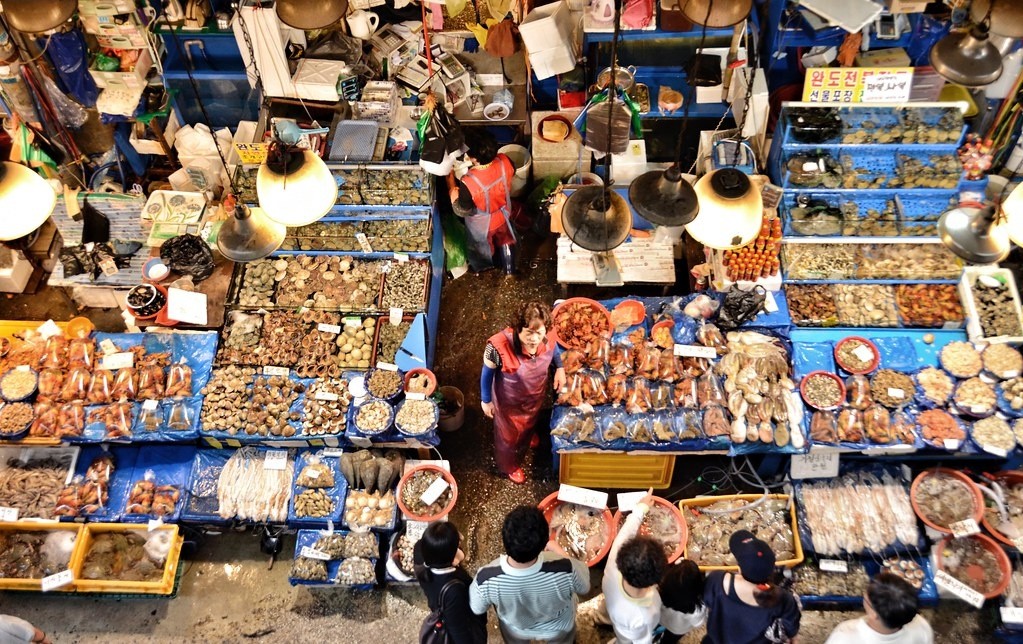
[0,337,11,356]
[0,402,35,436]
[914,340,1023,455]
[353,365,439,436]
[1,367,38,400]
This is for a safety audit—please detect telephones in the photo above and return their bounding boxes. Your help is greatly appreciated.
[370,26,408,54]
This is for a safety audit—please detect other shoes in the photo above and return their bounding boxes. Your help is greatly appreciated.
[509,468,525,484]
[530,433,540,448]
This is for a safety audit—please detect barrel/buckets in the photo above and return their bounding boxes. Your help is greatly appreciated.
[439,387,465,431]
[498,145,531,196]
[3,118,15,139]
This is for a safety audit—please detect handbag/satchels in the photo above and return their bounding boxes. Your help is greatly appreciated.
[419,579,467,644]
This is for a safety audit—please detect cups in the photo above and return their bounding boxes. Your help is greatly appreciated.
[96,4,133,47]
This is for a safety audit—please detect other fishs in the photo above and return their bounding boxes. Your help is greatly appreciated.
[216,445,295,524]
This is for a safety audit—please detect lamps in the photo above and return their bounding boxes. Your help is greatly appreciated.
[164,0,348,263]
[682,0,769,251]
[929,1,1023,90]
[1,0,77,33]
[0,160,56,241]
[561,0,635,252]
[628,0,713,226]
[936,155,1023,264]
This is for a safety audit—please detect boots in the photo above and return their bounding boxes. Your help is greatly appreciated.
[502,243,521,282]
[467,263,496,272]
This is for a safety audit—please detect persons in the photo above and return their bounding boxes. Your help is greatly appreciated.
[468,503,591,644]
[825,573,935,644]
[706,530,801,644]
[414,521,488,644]
[445,137,522,274]
[602,486,709,644]
[481,301,566,485]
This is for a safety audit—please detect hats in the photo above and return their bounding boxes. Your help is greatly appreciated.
[730,530,775,578]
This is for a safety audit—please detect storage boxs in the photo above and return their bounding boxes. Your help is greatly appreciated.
[694,46,770,175]
[0,445,186,599]
[0,243,33,293]
[958,266,1023,348]
[678,494,805,571]
[518,1,582,81]
[295,59,345,101]
[77,0,260,156]
[76,287,135,311]
[611,140,647,186]
[370,257,430,370]
[888,0,927,14]
[800,45,912,67]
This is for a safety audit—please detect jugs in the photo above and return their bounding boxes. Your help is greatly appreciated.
[346,11,379,41]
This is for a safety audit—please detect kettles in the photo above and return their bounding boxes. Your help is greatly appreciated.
[590,0,615,23]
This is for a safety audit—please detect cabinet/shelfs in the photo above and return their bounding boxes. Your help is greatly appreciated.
[77,0,180,178]
[232,1,306,98]
[581,6,760,117]
[768,101,968,284]
[765,1,924,80]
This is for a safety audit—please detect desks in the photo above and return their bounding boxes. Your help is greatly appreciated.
[134,245,234,327]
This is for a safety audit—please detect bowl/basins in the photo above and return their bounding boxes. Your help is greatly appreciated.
[660,92,684,110]
[537,490,614,567]
[127,257,170,319]
[568,172,604,187]
[910,467,1023,600]
[589,65,636,93]
[483,102,510,122]
[800,336,879,410]
[612,495,689,564]
[404,368,437,397]
[396,465,458,522]
[537,115,573,144]
[67,317,92,340]
